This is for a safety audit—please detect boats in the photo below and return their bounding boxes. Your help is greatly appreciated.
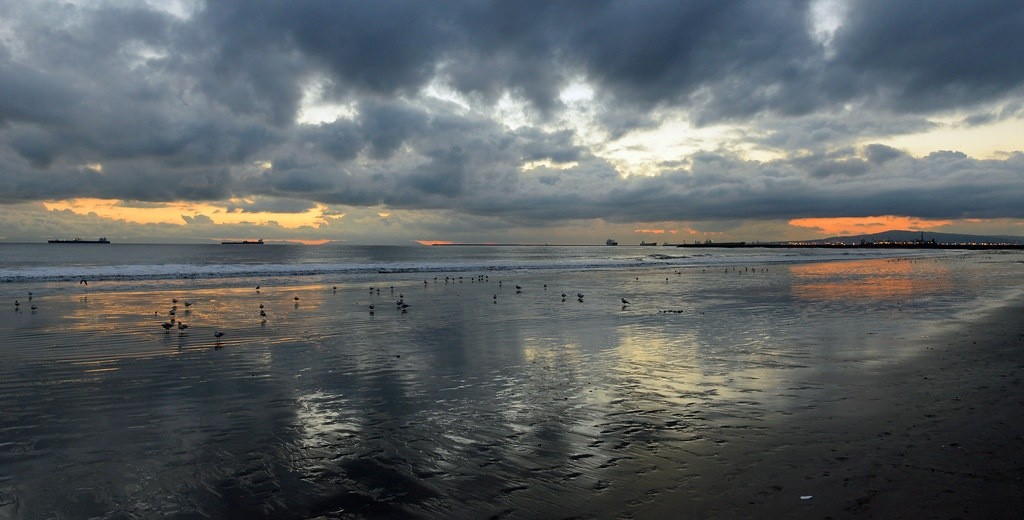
[222,238,265,244]
[47,236,110,243]
[640,240,657,246]
[606,239,618,246]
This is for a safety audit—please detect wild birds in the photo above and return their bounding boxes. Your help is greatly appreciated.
[471,274,489,282]
[444,275,463,282]
[14,300,20,307]
[332,285,337,289]
[259,303,266,325]
[499,281,502,284]
[423,279,428,284]
[433,276,437,280]
[256,285,260,289]
[368,304,374,317]
[390,285,410,314]
[635,266,770,282]
[160,299,192,331]
[294,296,299,300]
[561,292,566,297]
[31,304,37,309]
[493,294,497,299]
[28,291,33,296]
[544,283,547,288]
[577,293,584,298]
[80,279,88,285]
[369,287,381,295]
[214,330,225,339]
[621,297,631,306]
[515,285,522,290]
